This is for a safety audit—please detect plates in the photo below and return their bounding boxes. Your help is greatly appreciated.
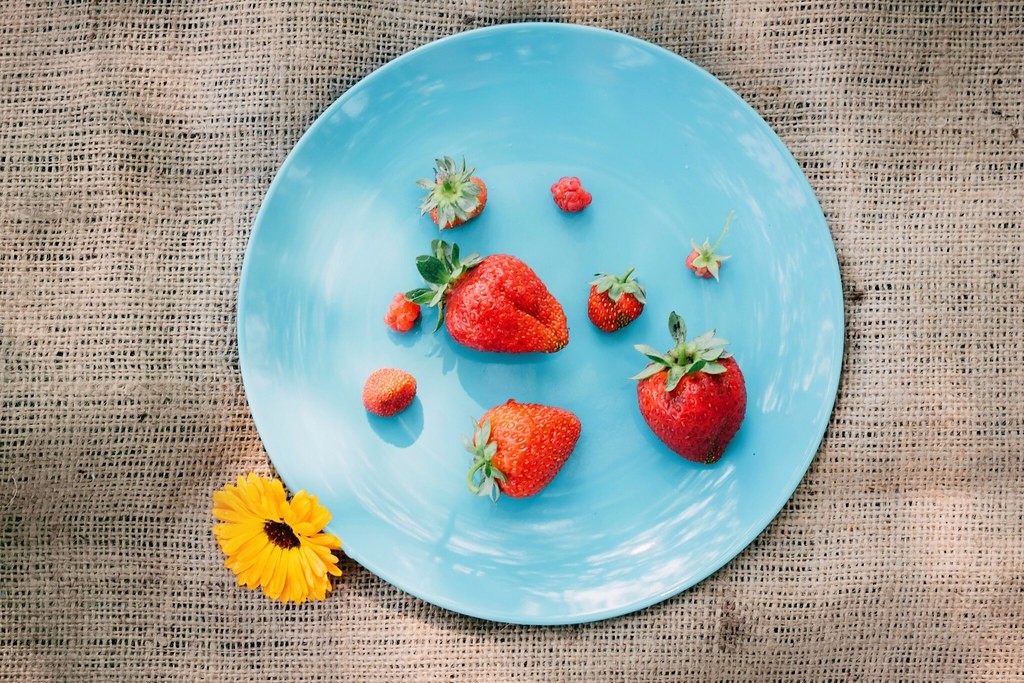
[236,23,845,631]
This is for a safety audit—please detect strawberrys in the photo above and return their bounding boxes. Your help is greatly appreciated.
[364,155,748,502]
